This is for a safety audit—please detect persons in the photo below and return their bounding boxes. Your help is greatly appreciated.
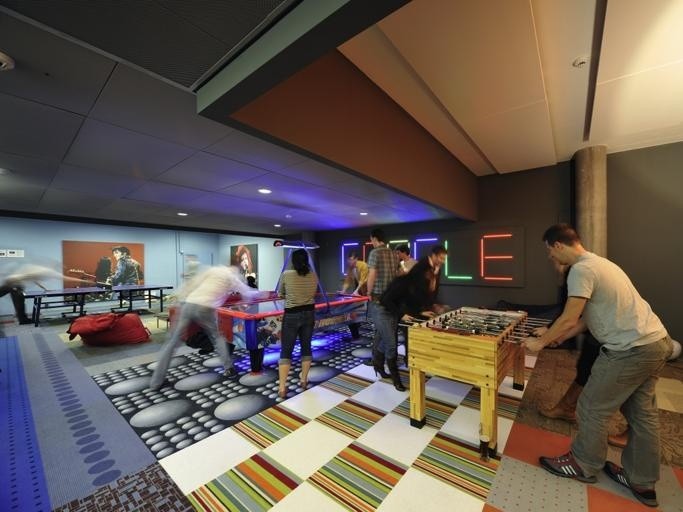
[365,226,403,303]
[0,259,95,326]
[391,244,420,280]
[334,249,371,301]
[178,253,201,281]
[91,255,116,285]
[401,242,450,368]
[371,261,437,391]
[520,223,674,508]
[276,248,319,399]
[530,227,630,447]
[105,245,144,300]
[149,262,275,393]
[230,244,254,276]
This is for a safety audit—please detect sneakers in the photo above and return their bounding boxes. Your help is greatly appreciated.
[539,452,598,484]
[225,367,237,377]
[20,318,33,325]
[602,461,659,506]
[149,386,160,392]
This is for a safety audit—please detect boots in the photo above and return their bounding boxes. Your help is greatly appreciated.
[373,350,391,378]
[608,424,629,448]
[539,381,584,421]
[387,358,405,392]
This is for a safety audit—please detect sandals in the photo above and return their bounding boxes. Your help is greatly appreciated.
[301,382,308,389]
[278,392,286,398]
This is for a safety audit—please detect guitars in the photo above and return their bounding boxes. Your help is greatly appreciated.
[67,269,144,285]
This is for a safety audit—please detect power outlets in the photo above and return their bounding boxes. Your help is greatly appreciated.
[0,250,6,257]
[7,250,23,257]
